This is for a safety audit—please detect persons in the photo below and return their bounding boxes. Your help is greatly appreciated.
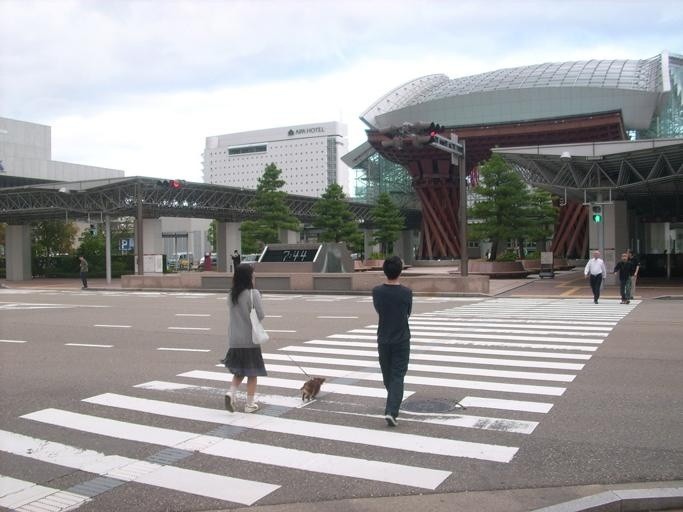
[220,263,265,411]
[372,256,412,426]
[626,248,641,300]
[614,253,632,304]
[78,255,88,288]
[230,250,240,273]
[584,250,606,304]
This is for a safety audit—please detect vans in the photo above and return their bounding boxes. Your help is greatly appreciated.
[168,252,193,270]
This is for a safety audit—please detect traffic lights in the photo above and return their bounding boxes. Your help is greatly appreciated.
[591,204,603,224]
[414,120,445,150]
[156,179,185,189]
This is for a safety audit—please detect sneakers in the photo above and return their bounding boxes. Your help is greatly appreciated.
[245,403,258,413]
[385,414,398,427]
[225,392,235,412]
[620,298,629,304]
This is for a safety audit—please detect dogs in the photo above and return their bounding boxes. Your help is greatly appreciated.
[298,377,326,402]
